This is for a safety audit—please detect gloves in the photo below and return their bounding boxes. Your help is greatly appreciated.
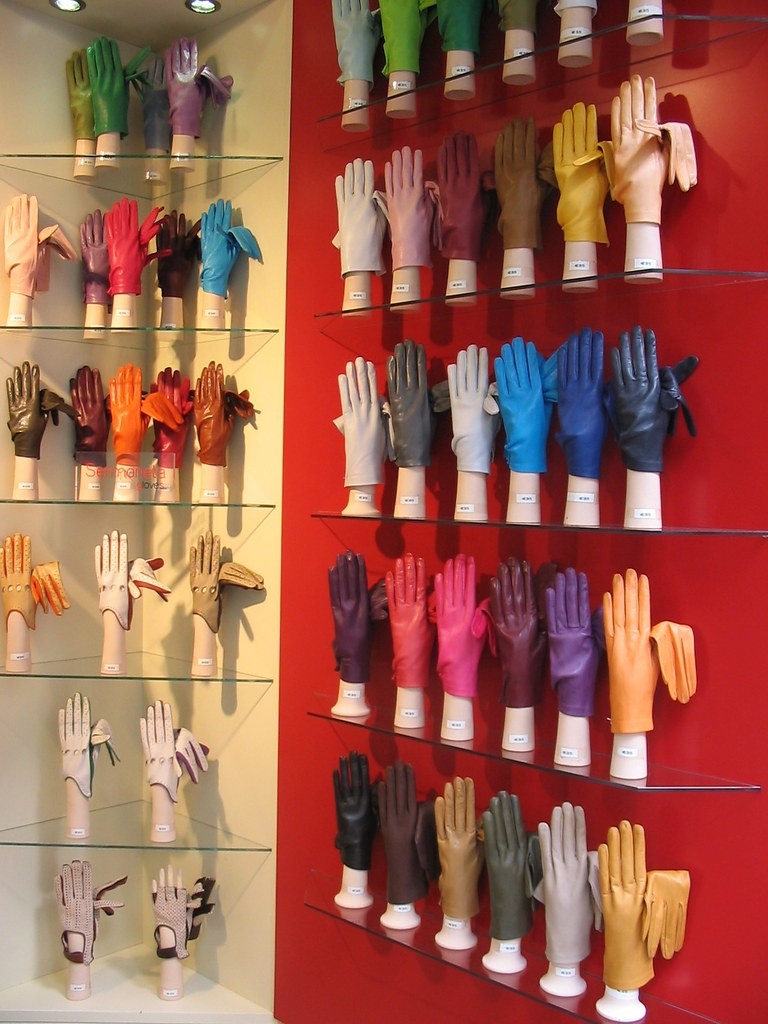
[334,325,699,489]
[140,700,209,803]
[0,530,264,633]
[6,361,253,467]
[328,549,697,734]
[331,74,698,279]
[66,37,234,152]
[332,750,690,991]
[331,0,438,91]
[58,692,121,797]
[54,860,128,966]
[5,194,262,313]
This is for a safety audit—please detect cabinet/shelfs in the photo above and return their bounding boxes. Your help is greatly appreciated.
[302,12,768,1024]
[0,153,284,1024]
